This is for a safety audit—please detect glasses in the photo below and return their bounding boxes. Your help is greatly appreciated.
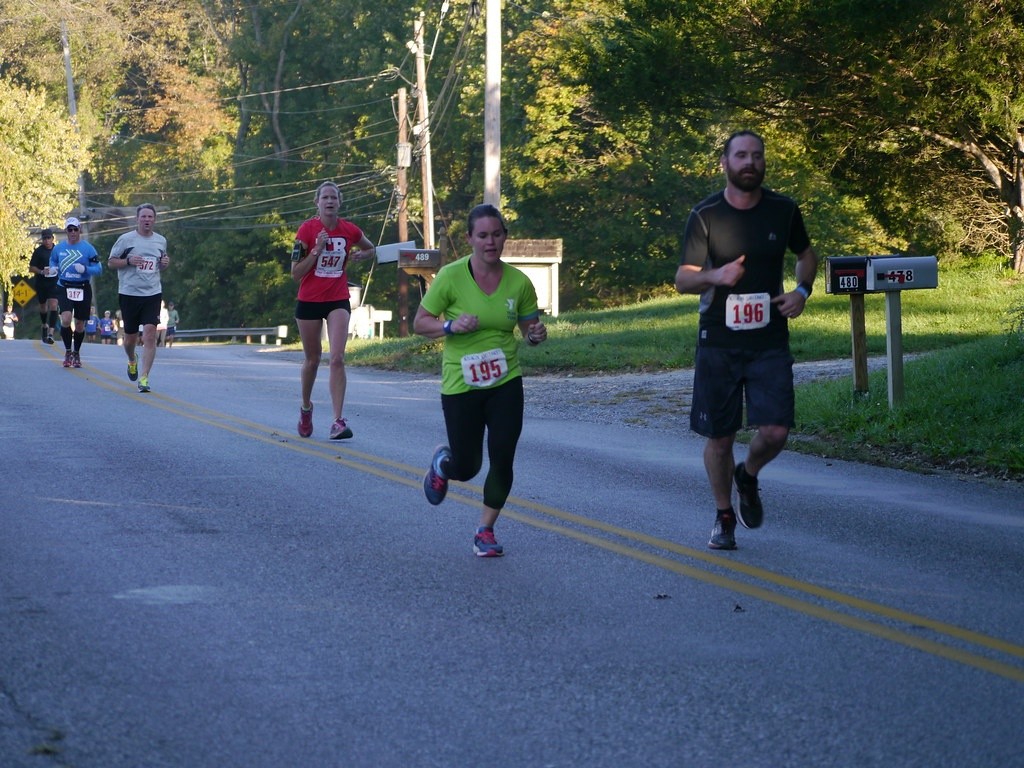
[67,227,79,232]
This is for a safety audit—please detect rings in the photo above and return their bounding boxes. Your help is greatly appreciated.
[464,318,469,322]
[790,312,792,317]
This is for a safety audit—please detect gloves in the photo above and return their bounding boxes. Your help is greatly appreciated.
[74,264,85,273]
[49,267,58,274]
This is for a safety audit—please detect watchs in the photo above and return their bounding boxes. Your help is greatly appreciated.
[311,248,319,258]
[444,320,454,334]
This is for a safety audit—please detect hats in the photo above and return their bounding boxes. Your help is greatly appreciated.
[42,229,53,238]
[65,217,80,229]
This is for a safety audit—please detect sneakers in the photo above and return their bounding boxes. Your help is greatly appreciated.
[138,377,150,392]
[473,525,504,558]
[329,417,354,439]
[297,402,313,437]
[72,352,81,367]
[734,462,763,528]
[63,351,72,366]
[707,508,737,547]
[127,353,138,381]
[424,445,452,505]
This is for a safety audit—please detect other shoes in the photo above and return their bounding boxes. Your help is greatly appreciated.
[42,324,49,343]
[47,337,54,344]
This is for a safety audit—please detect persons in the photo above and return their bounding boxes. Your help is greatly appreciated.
[108,203,168,392]
[49,217,102,368]
[0,303,19,341]
[57,299,178,350]
[414,203,548,556]
[29,229,58,344]
[290,182,375,440]
[675,132,819,549]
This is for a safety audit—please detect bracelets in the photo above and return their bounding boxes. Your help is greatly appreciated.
[796,287,809,299]
[126,257,131,266]
[41,270,45,275]
[527,332,538,344]
[795,280,813,294]
